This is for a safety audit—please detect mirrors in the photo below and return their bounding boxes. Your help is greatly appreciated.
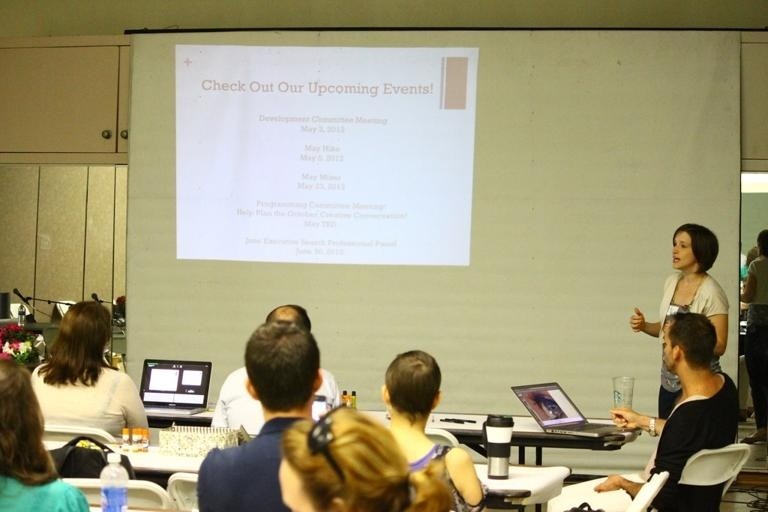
[738,171,768,475]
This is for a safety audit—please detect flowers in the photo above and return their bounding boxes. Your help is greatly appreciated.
[0,323,46,370]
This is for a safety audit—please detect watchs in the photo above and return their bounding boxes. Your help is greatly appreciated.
[646,416,657,437]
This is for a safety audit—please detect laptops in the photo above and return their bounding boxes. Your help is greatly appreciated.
[140,358,212,416]
[511,382,624,439]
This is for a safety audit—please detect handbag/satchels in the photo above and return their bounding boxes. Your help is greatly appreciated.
[49,437,136,479]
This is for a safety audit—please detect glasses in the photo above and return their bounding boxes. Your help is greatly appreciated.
[308,403,347,480]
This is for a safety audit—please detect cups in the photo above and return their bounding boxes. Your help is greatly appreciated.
[610,375,634,411]
[484,415,515,478]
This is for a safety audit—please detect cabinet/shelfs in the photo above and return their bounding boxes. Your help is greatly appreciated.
[0,45,129,166]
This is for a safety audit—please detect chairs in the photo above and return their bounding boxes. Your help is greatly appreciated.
[651,441,750,509]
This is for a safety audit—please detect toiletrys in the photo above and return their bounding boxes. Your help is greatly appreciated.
[348,390,358,409]
[341,389,349,405]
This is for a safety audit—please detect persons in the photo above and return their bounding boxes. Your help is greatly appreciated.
[29,299,149,441]
[0,357,89,512]
[629,222,730,422]
[196,318,324,512]
[276,402,458,511]
[379,350,486,512]
[592,311,741,511]
[739,228,767,444]
[210,303,342,438]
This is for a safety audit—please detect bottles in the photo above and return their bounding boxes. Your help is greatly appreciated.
[341,389,358,409]
[18,301,26,326]
[100,453,128,512]
[122,428,149,452]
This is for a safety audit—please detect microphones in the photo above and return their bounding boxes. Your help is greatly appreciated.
[92,293,104,302]
[12,288,31,306]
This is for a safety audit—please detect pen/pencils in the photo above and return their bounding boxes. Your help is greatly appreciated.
[445,418,476,423]
[440,419,464,424]
